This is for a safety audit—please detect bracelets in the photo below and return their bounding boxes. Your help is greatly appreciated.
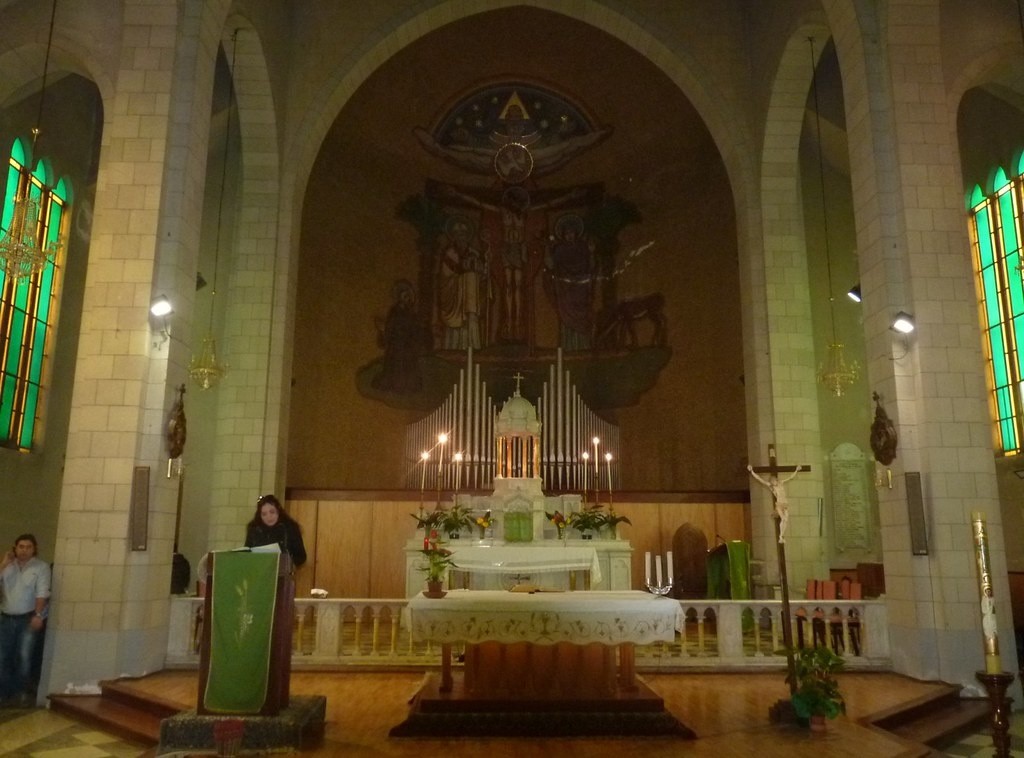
[35,612,44,620]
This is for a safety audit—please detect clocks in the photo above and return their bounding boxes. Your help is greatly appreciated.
[868,391,897,466]
[166,382,187,459]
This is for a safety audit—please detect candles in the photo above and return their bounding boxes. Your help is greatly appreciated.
[971,509,1003,674]
[418,435,462,492]
[642,551,675,599]
[582,437,613,491]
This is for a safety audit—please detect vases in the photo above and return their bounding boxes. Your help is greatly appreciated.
[557,526,566,538]
[478,526,486,539]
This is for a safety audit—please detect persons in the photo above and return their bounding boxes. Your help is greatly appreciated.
[243,494,307,566]
[171,543,191,594]
[380,286,434,405]
[747,464,802,544]
[0,533,52,709]
[543,222,592,353]
[431,222,496,351]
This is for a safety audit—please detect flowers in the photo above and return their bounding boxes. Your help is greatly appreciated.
[544,510,579,528]
[475,511,494,528]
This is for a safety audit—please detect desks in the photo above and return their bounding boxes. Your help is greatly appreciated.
[403,537,636,598]
[389,590,698,740]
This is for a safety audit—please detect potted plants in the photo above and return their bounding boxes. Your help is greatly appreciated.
[773,642,847,735]
[570,504,608,539]
[443,505,477,539]
[411,529,459,598]
[410,509,448,540]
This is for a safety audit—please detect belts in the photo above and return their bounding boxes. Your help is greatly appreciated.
[1,610,35,620]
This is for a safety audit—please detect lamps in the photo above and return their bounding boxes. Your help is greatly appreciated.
[890,311,915,335]
[193,271,208,293]
[185,26,238,393]
[0,0,58,285]
[799,34,862,400]
[845,282,862,305]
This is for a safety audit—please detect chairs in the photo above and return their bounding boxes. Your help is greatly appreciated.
[794,578,862,655]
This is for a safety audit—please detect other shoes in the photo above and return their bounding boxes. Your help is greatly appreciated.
[0,693,32,709]
